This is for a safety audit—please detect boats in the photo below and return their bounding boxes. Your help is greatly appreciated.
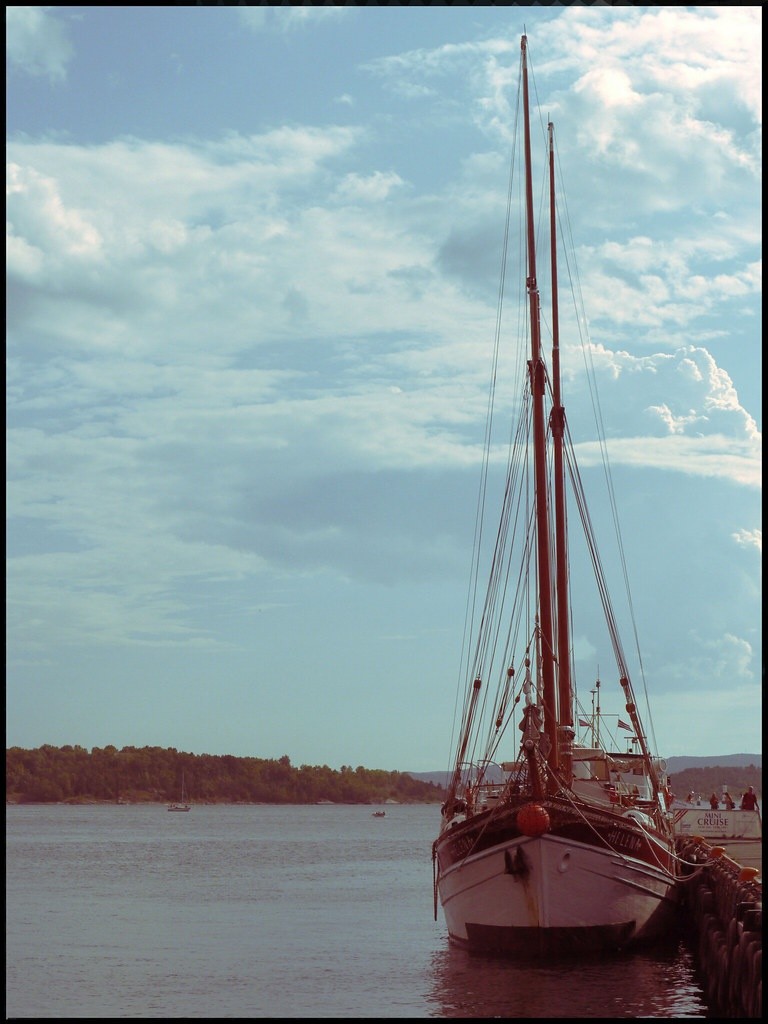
[373,811,386,817]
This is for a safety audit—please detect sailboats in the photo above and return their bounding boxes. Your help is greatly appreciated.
[166,768,191,812]
[429,31,691,971]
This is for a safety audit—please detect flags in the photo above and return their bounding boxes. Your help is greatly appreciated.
[618,719,633,732]
[579,718,593,728]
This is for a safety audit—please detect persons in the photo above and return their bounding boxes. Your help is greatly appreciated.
[721,792,734,811]
[709,792,720,810]
[740,786,761,810]
[684,791,703,806]
